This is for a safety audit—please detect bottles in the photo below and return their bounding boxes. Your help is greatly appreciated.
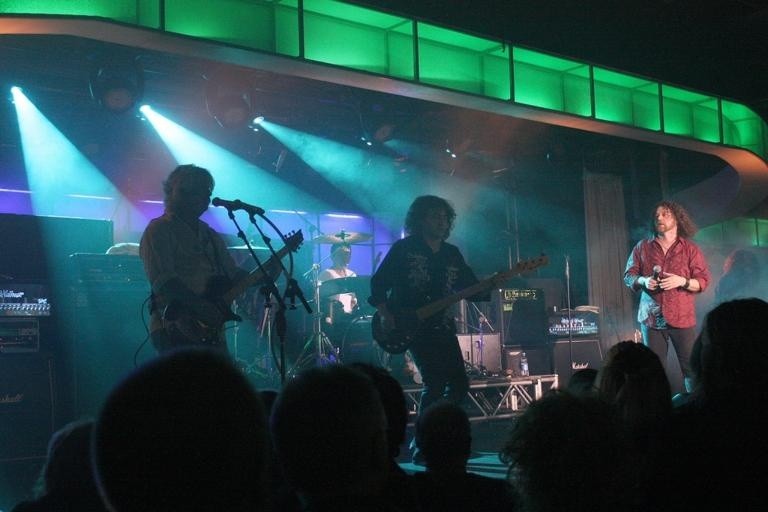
[521,353,529,377]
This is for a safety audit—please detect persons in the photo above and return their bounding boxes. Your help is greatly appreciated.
[138,165,283,360]
[368,196,504,465]
[714,249,760,302]
[13,297,768,512]
[623,200,712,392]
[318,242,358,323]
[106,243,140,256]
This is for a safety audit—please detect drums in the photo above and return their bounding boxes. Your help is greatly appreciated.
[342,316,405,374]
[320,300,343,327]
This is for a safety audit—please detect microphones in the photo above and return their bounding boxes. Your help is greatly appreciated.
[212,197,265,216]
[652,264,661,281]
[370,250,383,277]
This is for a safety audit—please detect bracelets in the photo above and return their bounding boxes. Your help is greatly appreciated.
[683,278,690,289]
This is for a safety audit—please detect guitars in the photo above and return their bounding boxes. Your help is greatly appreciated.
[163,230,304,358]
[372,254,548,354]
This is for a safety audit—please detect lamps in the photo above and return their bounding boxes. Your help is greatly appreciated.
[86,45,147,113]
[200,63,262,128]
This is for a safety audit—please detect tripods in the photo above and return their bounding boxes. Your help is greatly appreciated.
[286,242,342,378]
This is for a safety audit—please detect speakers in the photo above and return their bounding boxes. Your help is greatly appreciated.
[68,252,160,422]
[496,287,547,349]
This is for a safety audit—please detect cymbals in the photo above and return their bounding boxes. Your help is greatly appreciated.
[310,233,370,244]
[318,276,370,294]
